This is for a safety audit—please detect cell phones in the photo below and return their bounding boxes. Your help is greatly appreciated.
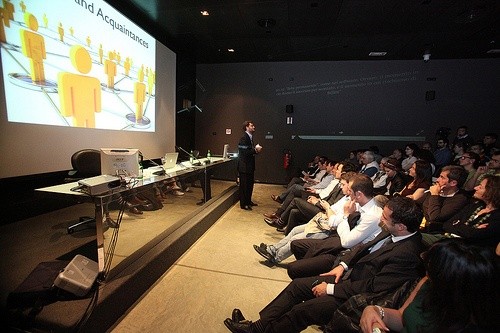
[312,280,320,287]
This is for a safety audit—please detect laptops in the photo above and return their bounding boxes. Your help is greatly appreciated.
[223,145,230,159]
[157,153,179,169]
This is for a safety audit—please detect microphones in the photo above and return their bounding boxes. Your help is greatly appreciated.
[180,147,201,165]
[195,105,202,112]
[139,154,165,174]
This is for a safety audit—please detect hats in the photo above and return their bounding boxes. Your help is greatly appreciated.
[382,158,401,171]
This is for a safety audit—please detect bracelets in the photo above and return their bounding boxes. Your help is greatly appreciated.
[326,207,331,211]
[374,304,384,320]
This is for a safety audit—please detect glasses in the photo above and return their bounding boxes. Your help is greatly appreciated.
[460,156,472,160]
[489,157,500,163]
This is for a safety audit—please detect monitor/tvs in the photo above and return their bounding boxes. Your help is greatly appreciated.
[100,148,140,178]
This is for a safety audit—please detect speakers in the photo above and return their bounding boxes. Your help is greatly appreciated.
[53,253,101,295]
[286,105,293,113]
[425,91,435,101]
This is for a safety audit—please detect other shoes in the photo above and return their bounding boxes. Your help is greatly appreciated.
[102,181,203,233]
[283,185,287,189]
[263,213,285,232]
[270,194,283,204]
[253,243,276,264]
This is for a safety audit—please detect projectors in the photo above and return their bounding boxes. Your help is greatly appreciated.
[78,175,121,196]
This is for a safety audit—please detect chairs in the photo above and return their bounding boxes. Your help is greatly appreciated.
[64,148,101,234]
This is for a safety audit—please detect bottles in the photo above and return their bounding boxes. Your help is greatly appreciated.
[190,150,194,163]
[137,163,143,179]
[207,150,211,160]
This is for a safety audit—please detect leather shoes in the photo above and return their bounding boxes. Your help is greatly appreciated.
[223,308,254,333]
[250,201,258,206]
[241,205,252,210]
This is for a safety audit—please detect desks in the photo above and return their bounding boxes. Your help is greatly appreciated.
[33,156,232,275]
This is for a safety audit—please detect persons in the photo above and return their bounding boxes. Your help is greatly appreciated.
[101,170,213,228]
[224,121,500,333]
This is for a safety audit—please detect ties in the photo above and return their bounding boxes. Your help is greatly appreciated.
[380,235,394,248]
